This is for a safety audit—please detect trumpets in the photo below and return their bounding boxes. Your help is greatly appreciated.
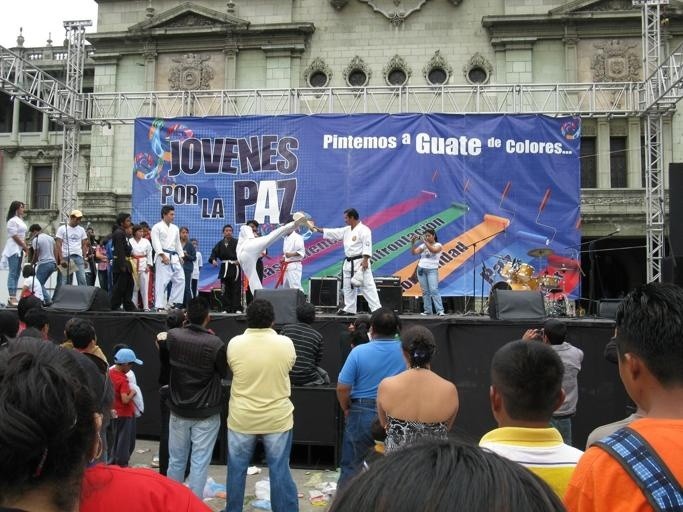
[410,232,427,241]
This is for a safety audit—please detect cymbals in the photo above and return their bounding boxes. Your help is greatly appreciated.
[554,268,578,272]
[527,249,554,257]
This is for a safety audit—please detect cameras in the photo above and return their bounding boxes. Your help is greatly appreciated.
[417,235,422,240]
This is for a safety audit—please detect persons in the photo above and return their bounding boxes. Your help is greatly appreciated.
[56,209,88,286]
[29,223,59,306]
[236,216,305,297]
[17,297,43,335]
[166,296,227,500]
[208,225,241,314]
[566,281,683,512]
[327,439,567,512]
[3,201,29,306]
[108,342,144,454]
[411,229,445,316]
[156,309,190,480]
[190,238,203,298]
[310,207,383,315]
[1,309,18,340]
[24,307,50,338]
[477,339,592,502]
[150,205,186,313]
[0,336,101,512]
[58,318,108,371]
[167,225,197,309]
[82,212,153,311]
[604,324,637,417]
[280,219,305,294]
[60,323,106,467]
[339,312,371,374]
[221,299,299,512]
[335,307,408,489]
[246,220,267,306]
[522,318,584,446]
[278,302,330,386]
[79,353,211,512]
[19,265,45,305]
[376,323,459,461]
[107,349,143,467]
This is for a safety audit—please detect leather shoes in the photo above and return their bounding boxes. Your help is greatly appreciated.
[8,298,19,307]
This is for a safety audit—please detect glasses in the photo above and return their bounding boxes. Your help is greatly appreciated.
[80,351,110,426]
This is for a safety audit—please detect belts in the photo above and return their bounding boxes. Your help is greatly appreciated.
[351,398,376,404]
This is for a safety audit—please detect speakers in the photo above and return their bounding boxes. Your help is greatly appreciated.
[598,302,621,319]
[490,288,547,321]
[51,284,108,311]
[252,288,305,324]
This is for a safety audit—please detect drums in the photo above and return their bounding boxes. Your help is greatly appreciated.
[538,274,560,291]
[529,276,545,289]
[500,261,516,277]
[489,280,532,291]
[515,263,535,282]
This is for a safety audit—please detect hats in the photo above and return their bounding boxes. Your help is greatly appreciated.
[113,348,143,366]
[70,210,83,218]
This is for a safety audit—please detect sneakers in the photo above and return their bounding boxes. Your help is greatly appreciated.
[337,309,355,315]
[437,311,445,316]
[41,300,52,308]
[421,311,433,316]
